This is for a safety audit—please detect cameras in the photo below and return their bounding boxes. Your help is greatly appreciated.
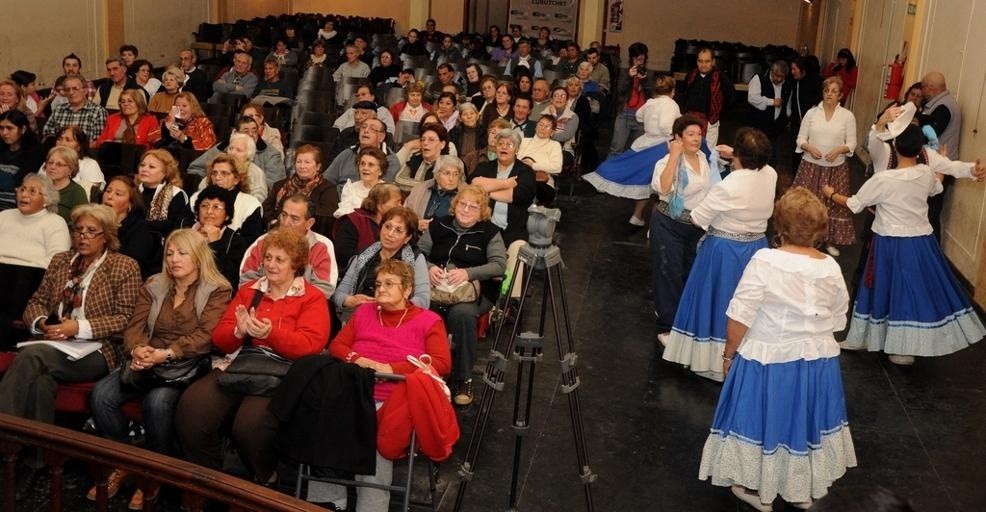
[636,65,646,74]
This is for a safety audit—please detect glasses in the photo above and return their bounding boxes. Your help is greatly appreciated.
[373,279,402,288]
[70,230,105,239]
[16,184,45,199]
[199,202,225,211]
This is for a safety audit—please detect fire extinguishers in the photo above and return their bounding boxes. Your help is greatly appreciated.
[884,54,907,100]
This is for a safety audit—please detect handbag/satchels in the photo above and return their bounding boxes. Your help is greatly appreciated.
[430,265,481,304]
[119,350,212,389]
[215,346,295,396]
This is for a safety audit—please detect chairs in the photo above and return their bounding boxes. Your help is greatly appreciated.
[0,12,620,512]
[671,39,801,83]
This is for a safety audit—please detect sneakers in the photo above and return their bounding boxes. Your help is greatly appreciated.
[790,498,813,509]
[628,216,645,226]
[656,333,668,346]
[452,378,473,405]
[731,486,772,512]
[890,356,914,365]
[826,247,840,257]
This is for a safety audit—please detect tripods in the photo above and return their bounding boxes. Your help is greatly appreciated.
[452,255,597,511]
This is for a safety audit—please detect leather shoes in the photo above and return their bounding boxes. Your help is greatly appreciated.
[14,464,46,499]
[128,477,160,510]
[502,305,520,323]
[85,467,135,501]
[127,420,146,445]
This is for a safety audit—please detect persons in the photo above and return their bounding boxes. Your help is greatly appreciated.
[651,114,722,347]
[867,72,985,248]
[699,186,857,511]
[663,131,778,382]
[0,14,608,511]
[796,76,857,256]
[822,125,985,364]
[582,44,858,237]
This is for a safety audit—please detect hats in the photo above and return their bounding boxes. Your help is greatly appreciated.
[352,101,377,112]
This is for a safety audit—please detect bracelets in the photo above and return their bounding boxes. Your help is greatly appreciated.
[722,355,735,360]
